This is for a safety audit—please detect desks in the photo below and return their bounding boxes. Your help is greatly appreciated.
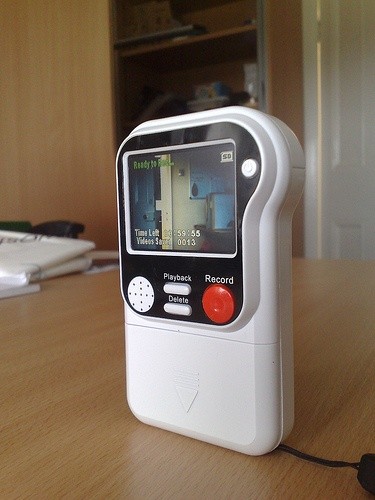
[0,255,375,500]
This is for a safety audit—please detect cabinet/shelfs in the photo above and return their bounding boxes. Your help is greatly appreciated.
[1,1,306,255]
